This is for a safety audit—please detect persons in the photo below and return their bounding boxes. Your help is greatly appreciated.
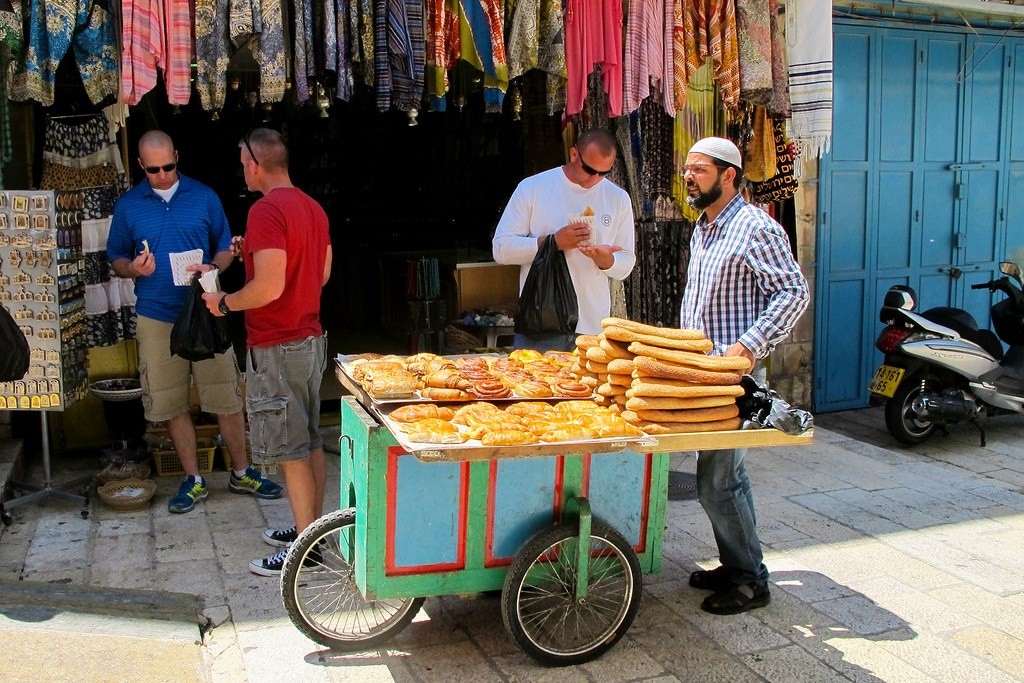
[104,132,285,513]
[199,129,333,577]
[490,131,637,357]
[680,137,809,618]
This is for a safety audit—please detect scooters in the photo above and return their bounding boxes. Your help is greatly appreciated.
[868,261,1024,449]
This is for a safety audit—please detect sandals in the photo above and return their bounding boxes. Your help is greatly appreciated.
[689,562,740,589]
[702,576,771,614]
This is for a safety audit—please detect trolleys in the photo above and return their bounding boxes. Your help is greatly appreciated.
[279,355,814,668]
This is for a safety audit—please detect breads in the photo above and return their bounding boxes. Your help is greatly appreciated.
[234,236,244,255]
[352,348,644,446]
[570,318,751,434]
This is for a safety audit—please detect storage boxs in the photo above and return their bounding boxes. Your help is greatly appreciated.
[154,437,217,474]
[220,440,279,470]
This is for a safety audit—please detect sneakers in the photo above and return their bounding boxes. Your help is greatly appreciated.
[262,525,329,552]
[249,536,324,575]
[167,477,209,513]
[228,467,283,499]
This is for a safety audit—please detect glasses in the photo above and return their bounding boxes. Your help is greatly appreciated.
[141,154,177,174]
[575,144,613,177]
[244,131,259,166]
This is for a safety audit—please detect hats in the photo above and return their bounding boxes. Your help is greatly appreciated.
[689,137,742,170]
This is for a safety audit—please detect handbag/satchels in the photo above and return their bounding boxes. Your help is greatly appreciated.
[513,234,578,337]
[168,274,232,362]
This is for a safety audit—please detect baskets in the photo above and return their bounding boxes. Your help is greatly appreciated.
[220,436,256,472]
[99,480,158,513]
[152,435,217,474]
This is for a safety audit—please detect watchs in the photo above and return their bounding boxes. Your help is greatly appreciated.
[218,295,230,314]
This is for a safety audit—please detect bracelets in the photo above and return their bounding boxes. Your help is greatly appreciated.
[210,262,220,272]
